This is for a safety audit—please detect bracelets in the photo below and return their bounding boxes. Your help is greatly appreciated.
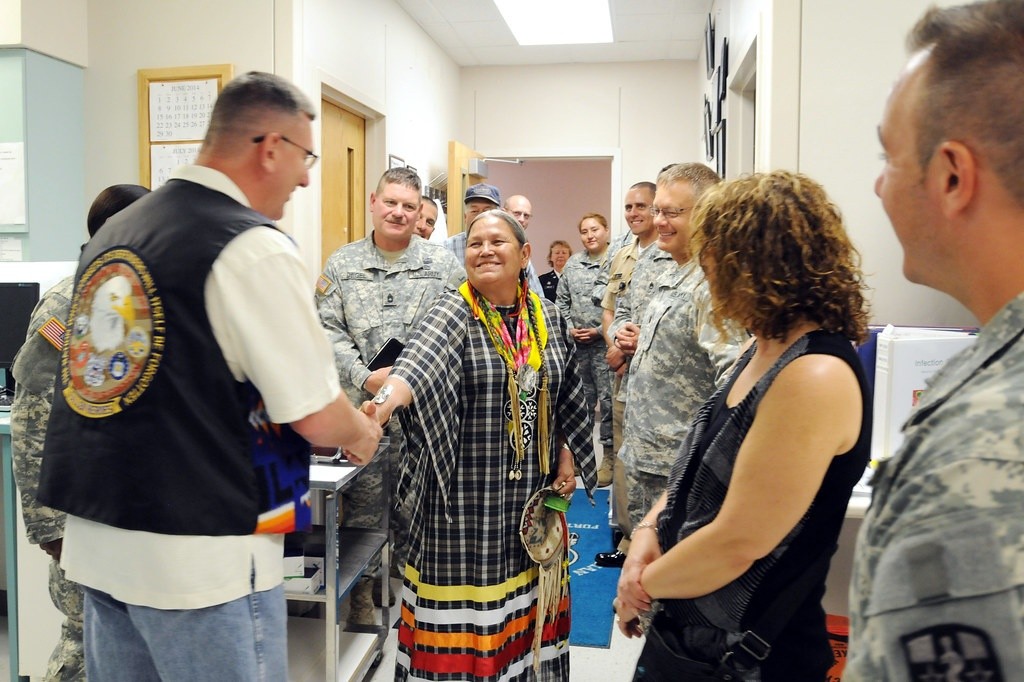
[560,441,571,451]
[630,521,660,540]
[371,384,397,430]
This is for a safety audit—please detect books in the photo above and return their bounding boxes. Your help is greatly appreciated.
[854,323,981,465]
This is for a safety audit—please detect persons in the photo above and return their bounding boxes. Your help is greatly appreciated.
[556,162,753,638]
[842,0,1024,682]
[11,184,153,682]
[316,169,467,633]
[412,197,439,241]
[441,183,572,303]
[616,171,865,682]
[39,74,382,682]
[344,212,578,682]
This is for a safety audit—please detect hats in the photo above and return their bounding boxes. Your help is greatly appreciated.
[463,184,501,207]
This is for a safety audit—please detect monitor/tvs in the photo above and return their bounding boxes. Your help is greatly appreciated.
[0,283,40,397]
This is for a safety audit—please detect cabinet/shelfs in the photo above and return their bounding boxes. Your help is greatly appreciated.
[286,435,393,682]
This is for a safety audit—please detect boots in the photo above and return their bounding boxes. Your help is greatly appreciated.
[346,580,376,628]
[372,576,397,608]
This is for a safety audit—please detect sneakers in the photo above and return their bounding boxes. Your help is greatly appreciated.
[594,448,615,486]
[572,457,580,476]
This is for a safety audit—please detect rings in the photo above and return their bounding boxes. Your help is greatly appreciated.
[615,614,620,622]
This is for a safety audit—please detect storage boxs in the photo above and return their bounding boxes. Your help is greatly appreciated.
[566,488,620,649]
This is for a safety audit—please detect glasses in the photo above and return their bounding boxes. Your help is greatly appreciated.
[649,205,693,218]
[253,133,320,171]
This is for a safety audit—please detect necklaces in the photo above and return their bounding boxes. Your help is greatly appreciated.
[488,309,538,479]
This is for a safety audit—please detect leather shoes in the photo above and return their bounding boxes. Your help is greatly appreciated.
[594,551,626,568]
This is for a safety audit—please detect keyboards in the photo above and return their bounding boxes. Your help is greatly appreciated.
[0,398,15,412]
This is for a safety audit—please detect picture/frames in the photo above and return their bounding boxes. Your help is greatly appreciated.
[389,154,405,169]
[702,12,727,180]
